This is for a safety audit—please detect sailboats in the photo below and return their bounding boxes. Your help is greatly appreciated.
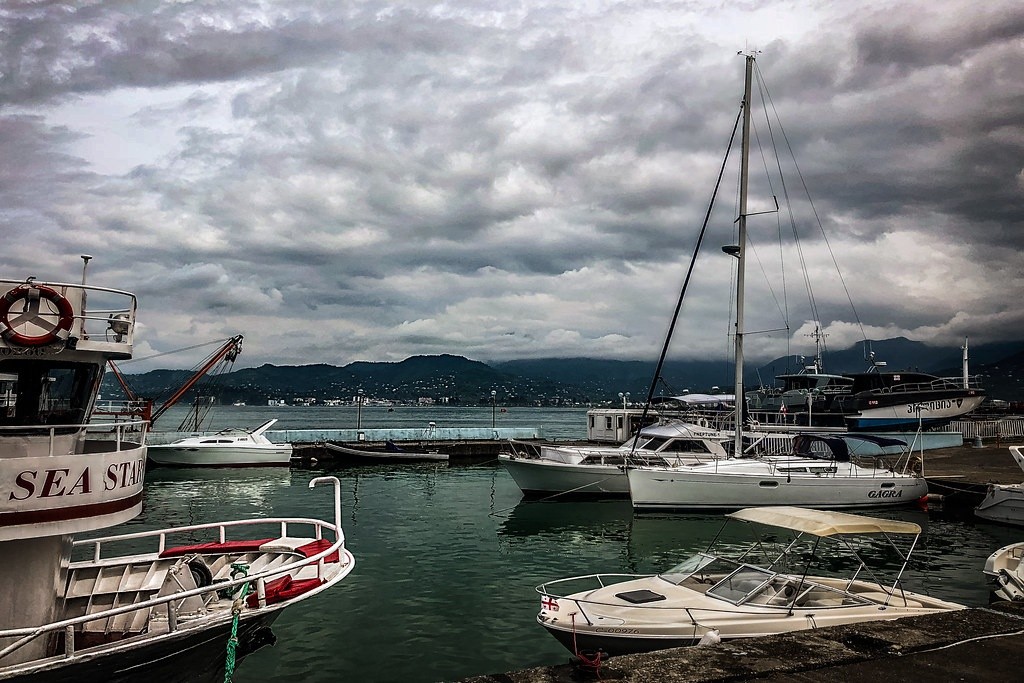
[625,50,931,516]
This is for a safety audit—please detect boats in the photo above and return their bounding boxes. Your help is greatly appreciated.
[536,504,971,666]
[679,368,989,424]
[148,417,294,468]
[586,391,963,455]
[0,254,354,683]
[499,418,840,505]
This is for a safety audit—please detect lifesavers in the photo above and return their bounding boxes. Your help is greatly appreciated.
[0,283,76,346]
